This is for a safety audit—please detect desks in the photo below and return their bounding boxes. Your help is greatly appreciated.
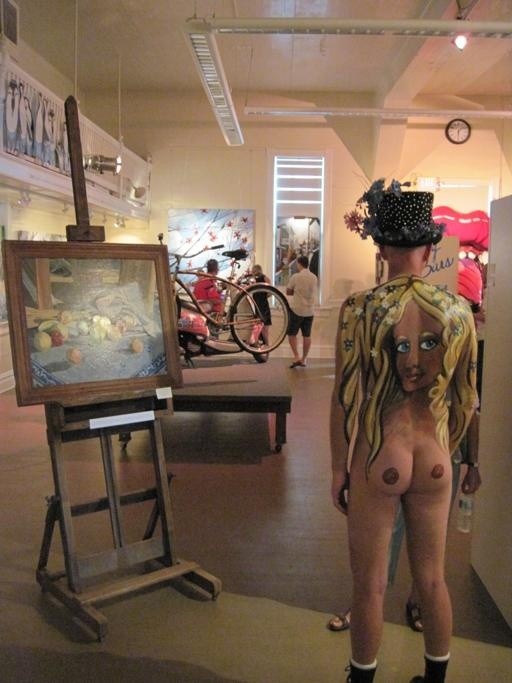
[118,364,293,452]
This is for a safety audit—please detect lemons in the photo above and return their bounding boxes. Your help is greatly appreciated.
[33,331,52,351]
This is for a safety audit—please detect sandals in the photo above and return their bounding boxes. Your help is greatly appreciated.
[290,361,302,368]
[330,608,352,631]
[406,597,424,632]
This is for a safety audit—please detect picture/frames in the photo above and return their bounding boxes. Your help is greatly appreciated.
[1,237,184,409]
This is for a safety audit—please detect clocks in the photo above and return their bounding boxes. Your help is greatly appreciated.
[445,118,471,144]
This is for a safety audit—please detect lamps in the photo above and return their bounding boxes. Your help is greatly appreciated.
[88,154,122,174]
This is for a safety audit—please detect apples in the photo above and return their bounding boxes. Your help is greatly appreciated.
[51,331,65,347]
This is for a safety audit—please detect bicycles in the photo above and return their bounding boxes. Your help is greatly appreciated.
[159,233,290,353]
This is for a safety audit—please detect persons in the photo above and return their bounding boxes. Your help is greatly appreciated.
[188,259,273,348]
[328,192,489,682]
[287,256,318,368]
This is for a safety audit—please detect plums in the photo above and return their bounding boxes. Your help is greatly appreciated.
[65,349,83,363]
[131,339,144,352]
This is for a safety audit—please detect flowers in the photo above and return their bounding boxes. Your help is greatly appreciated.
[342,177,411,240]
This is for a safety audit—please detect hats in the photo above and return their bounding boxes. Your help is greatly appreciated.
[369,191,445,248]
